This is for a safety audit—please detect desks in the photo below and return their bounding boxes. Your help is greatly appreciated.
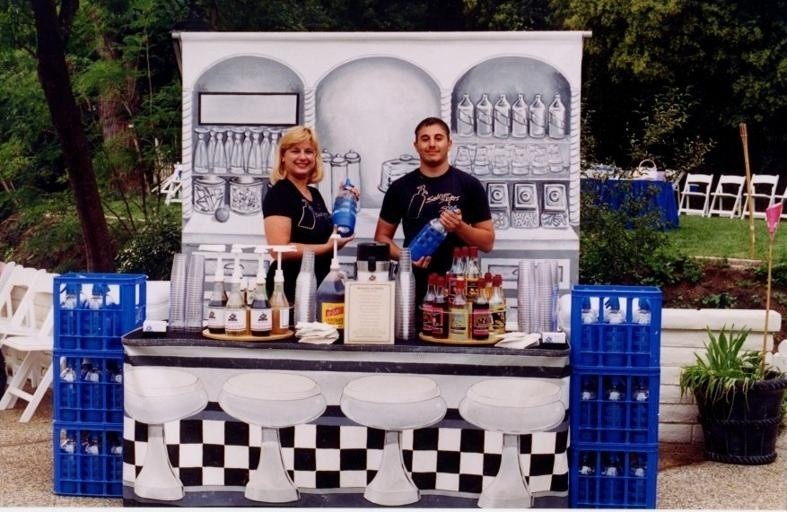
[580,178,679,232]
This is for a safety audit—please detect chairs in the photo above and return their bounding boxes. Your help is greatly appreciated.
[671,170,685,208]
[677,173,713,217]
[0,283,92,424]
[741,174,779,222]
[774,188,787,220]
[708,174,746,219]
[0,266,45,410]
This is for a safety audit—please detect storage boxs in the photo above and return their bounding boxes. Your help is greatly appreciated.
[53,273,147,353]
[570,285,663,371]
[568,445,659,509]
[570,370,660,448]
[53,423,124,498]
[52,349,124,426]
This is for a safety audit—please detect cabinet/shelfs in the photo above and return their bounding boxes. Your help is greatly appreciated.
[181,31,582,332]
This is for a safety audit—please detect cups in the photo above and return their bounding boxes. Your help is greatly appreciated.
[394,248,417,339]
[294,246,317,326]
[168,253,204,329]
[193,124,286,174]
[517,260,559,332]
[452,143,565,176]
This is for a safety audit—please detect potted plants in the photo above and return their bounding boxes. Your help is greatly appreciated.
[680,324,786,463]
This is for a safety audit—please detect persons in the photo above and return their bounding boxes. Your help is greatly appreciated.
[263,126,356,308]
[374,117,494,332]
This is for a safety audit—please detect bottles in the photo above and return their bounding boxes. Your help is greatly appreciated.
[511,94,528,140]
[445,246,462,283]
[331,179,359,238]
[193,126,297,176]
[468,246,480,278]
[461,246,470,279]
[249,269,274,337]
[529,92,547,139]
[485,272,492,300]
[407,204,459,262]
[491,274,507,336]
[493,93,511,140]
[470,278,492,340]
[316,259,345,344]
[449,279,468,339]
[433,276,449,340]
[423,274,436,335]
[548,94,567,141]
[475,91,493,138]
[456,93,476,138]
[269,268,290,335]
[224,272,250,337]
[207,281,228,334]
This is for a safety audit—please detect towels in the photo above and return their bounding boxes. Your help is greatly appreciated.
[495,330,541,350]
[295,321,338,346]
